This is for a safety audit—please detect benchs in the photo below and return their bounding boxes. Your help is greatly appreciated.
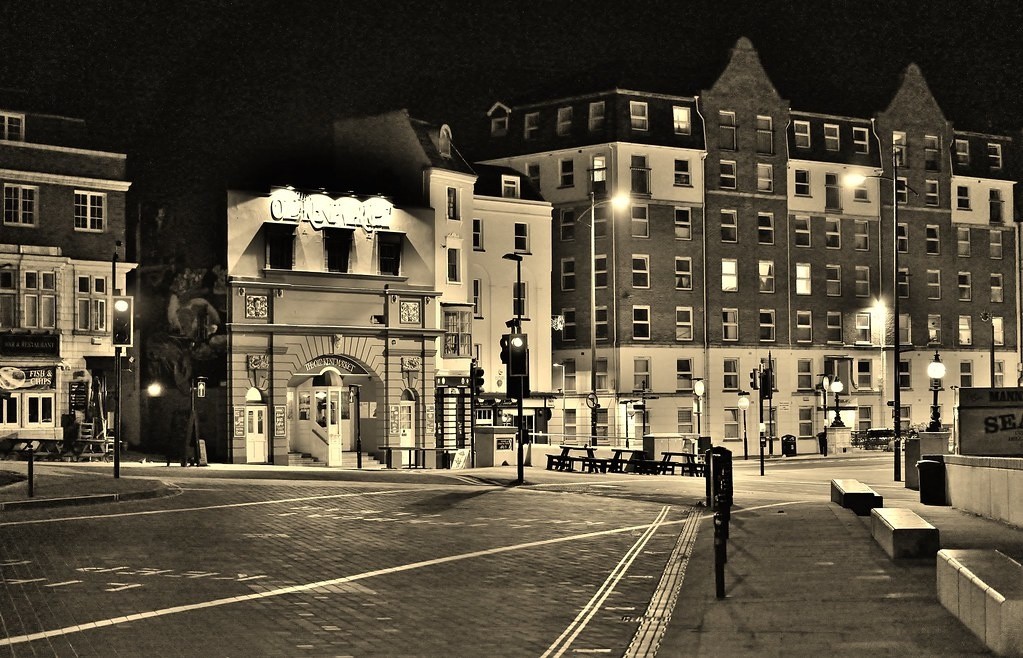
[831,478,883,516]
[545,454,706,477]
[937,549,1023,658]
[871,507,940,559]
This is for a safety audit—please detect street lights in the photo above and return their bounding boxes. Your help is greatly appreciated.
[577,190,631,447]
[843,147,918,482]
[830,377,845,427]
[502,254,524,483]
[925,350,946,432]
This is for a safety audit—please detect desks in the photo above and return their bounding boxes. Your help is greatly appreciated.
[657,452,699,477]
[377,446,458,469]
[5,438,103,461]
[555,445,599,473]
[607,449,650,475]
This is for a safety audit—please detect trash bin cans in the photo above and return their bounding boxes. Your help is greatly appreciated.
[781,434,796,456]
[916,460,945,504]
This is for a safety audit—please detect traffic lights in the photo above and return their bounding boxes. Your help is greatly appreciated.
[509,333,528,378]
[750,369,760,390]
[112,296,134,348]
[499,333,509,364]
